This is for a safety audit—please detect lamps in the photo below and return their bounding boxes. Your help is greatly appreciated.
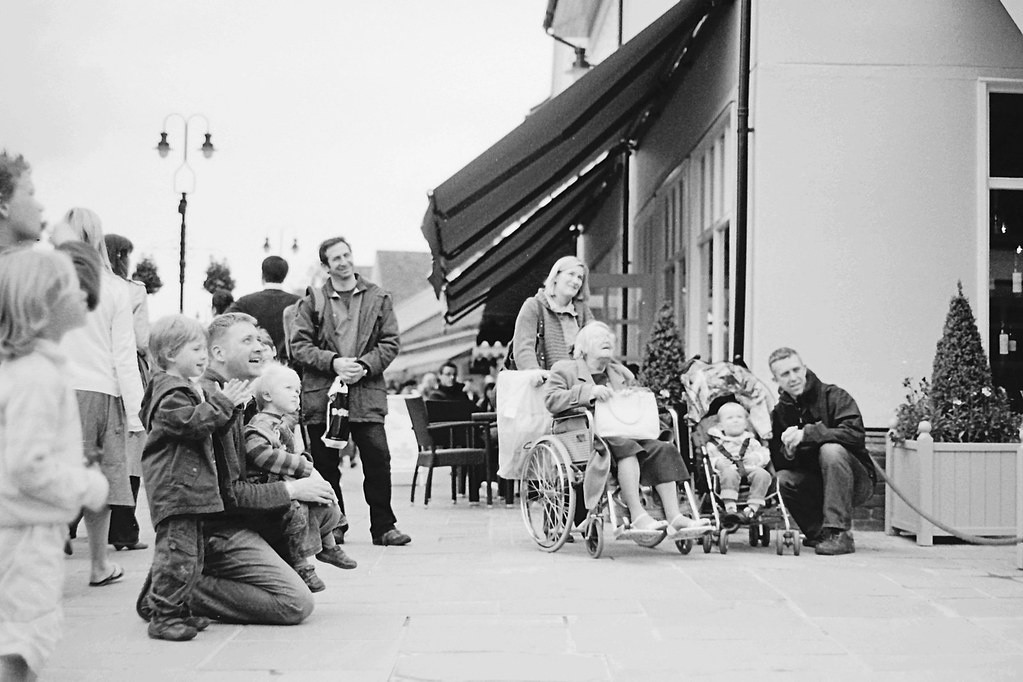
[543,19,591,69]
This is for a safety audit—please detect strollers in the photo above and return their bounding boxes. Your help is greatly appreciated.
[679,355,802,555]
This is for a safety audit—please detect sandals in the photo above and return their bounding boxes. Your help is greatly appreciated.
[725,505,756,524]
[666,514,711,536]
[629,514,668,531]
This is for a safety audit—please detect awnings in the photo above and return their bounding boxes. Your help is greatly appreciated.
[419,1,728,345]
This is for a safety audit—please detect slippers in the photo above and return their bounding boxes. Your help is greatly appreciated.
[89,566,124,587]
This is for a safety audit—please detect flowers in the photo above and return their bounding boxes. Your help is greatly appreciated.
[887,280,1023,449]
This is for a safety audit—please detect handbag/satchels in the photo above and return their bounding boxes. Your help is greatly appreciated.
[496,369,554,481]
[503,299,544,370]
[321,375,349,450]
[593,387,661,441]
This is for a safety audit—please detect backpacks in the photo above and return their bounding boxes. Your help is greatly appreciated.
[283,284,325,370]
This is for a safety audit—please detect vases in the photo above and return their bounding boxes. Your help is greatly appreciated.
[885,429,1023,546]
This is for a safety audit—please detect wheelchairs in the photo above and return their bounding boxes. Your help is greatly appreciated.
[519,401,711,555]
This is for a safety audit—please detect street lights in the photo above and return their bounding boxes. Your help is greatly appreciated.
[155,111,218,313]
[261,228,301,260]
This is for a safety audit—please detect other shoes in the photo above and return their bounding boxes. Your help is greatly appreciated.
[147,615,208,641]
[803,529,855,555]
[114,543,149,551]
[294,565,325,592]
[314,547,357,570]
[64,540,73,556]
[333,528,344,545]
[372,527,411,546]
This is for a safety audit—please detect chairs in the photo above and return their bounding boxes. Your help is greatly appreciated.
[404,396,489,506]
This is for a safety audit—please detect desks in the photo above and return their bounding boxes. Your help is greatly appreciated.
[471,411,519,504]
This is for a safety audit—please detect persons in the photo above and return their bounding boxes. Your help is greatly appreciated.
[707,403,772,524]
[138,313,255,640]
[385,362,504,501]
[512,256,600,543]
[0,155,352,682]
[291,237,411,547]
[545,321,711,530]
[768,346,876,555]
